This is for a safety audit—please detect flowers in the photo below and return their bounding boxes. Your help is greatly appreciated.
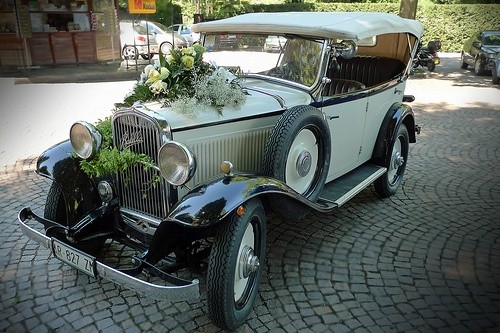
[112,44,253,120]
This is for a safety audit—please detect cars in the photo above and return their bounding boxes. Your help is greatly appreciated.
[459,29,500,76]
[167,23,207,49]
[264,35,288,53]
[119,21,160,60]
[140,19,189,56]
[216,34,241,51]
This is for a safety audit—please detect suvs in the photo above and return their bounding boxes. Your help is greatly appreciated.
[18,13,426,333]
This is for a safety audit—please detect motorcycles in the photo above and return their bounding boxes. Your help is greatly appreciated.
[414,39,442,71]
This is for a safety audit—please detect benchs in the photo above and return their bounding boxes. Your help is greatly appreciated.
[266,55,406,97]
